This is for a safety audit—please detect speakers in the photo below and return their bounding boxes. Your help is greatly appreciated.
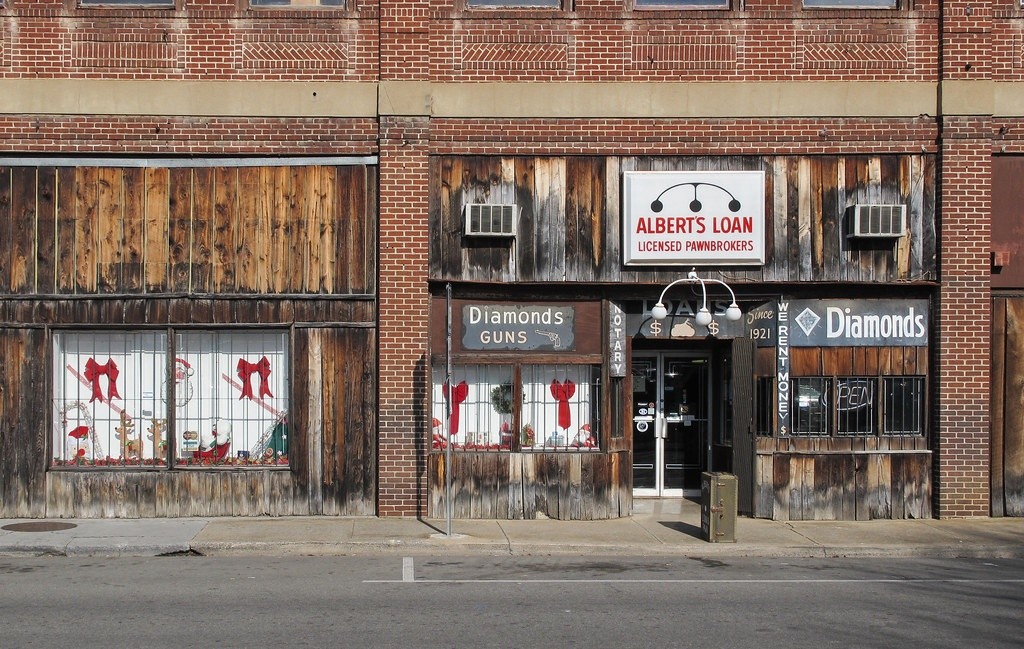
[701,471,738,543]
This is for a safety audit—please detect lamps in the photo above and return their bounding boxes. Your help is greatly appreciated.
[651,273,742,324]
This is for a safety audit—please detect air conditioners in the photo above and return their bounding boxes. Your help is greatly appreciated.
[462,203,518,238]
[847,204,907,238]
